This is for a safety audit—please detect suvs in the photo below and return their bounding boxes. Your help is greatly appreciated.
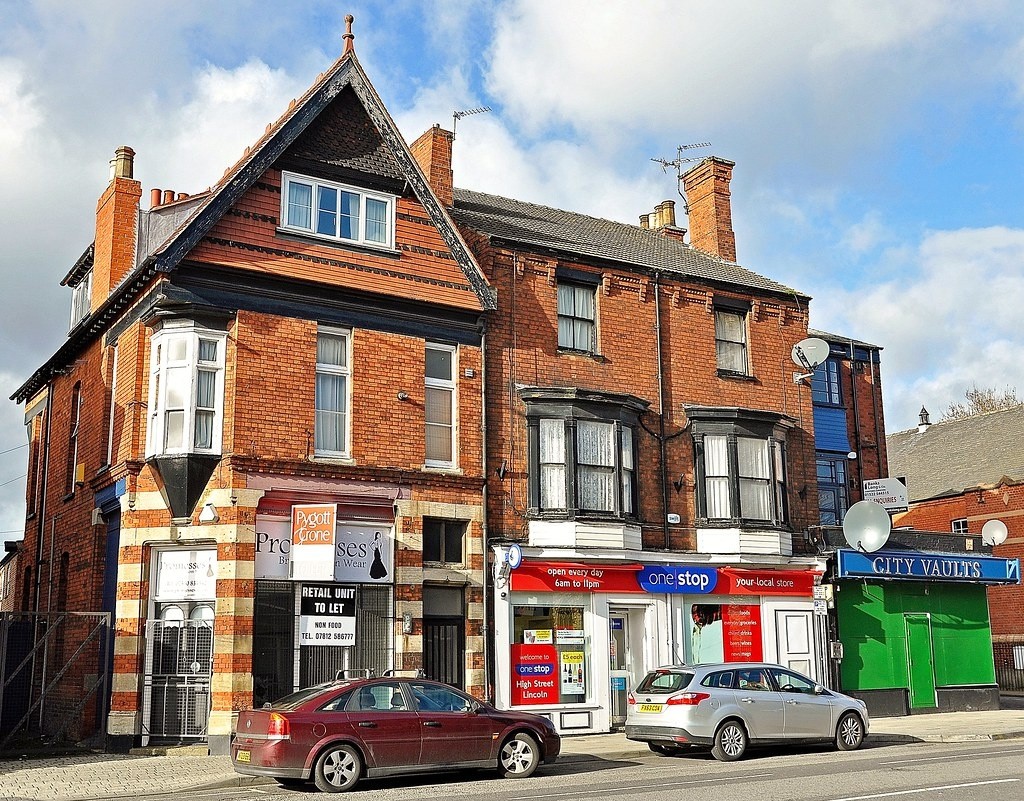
[624,661,870,762]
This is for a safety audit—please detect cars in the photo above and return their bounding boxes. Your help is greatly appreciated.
[229,673,560,795]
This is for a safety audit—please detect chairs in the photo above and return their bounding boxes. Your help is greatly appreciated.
[357,695,378,714]
[721,674,736,688]
[743,672,768,689]
[389,692,405,711]
